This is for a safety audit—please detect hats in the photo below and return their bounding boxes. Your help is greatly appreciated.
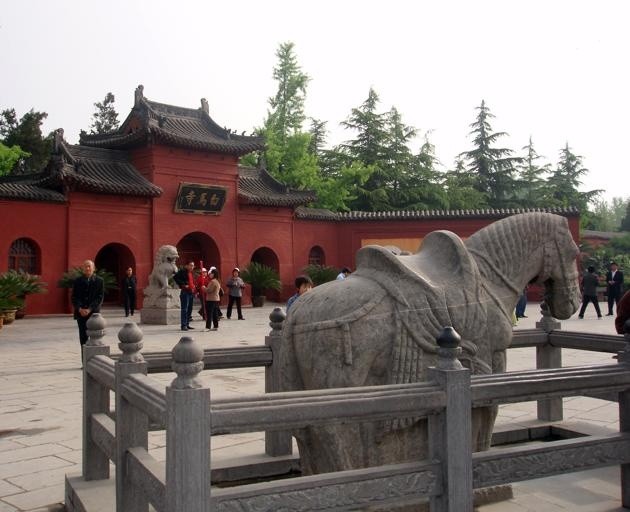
[208,266,217,274]
[233,267,240,272]
[202,267,208,272]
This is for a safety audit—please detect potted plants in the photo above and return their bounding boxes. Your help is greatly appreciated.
[240,262,280,307]
[0,270,49,331]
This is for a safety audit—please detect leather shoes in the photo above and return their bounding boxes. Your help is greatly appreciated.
[182,326,195,331]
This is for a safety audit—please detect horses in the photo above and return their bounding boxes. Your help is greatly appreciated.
[278,212,585,476]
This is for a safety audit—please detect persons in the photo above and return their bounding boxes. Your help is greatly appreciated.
[195,264,224,333]
[71,259,106,370]
[336,266,352,281]
[225,267,247,320]
[514,287,528,319]
[284,274,316,315]
[120,266,137,316]
[614,289,630,334]
[172,259,197,332]
[605,259,624,317]
[578,265,604,319]
